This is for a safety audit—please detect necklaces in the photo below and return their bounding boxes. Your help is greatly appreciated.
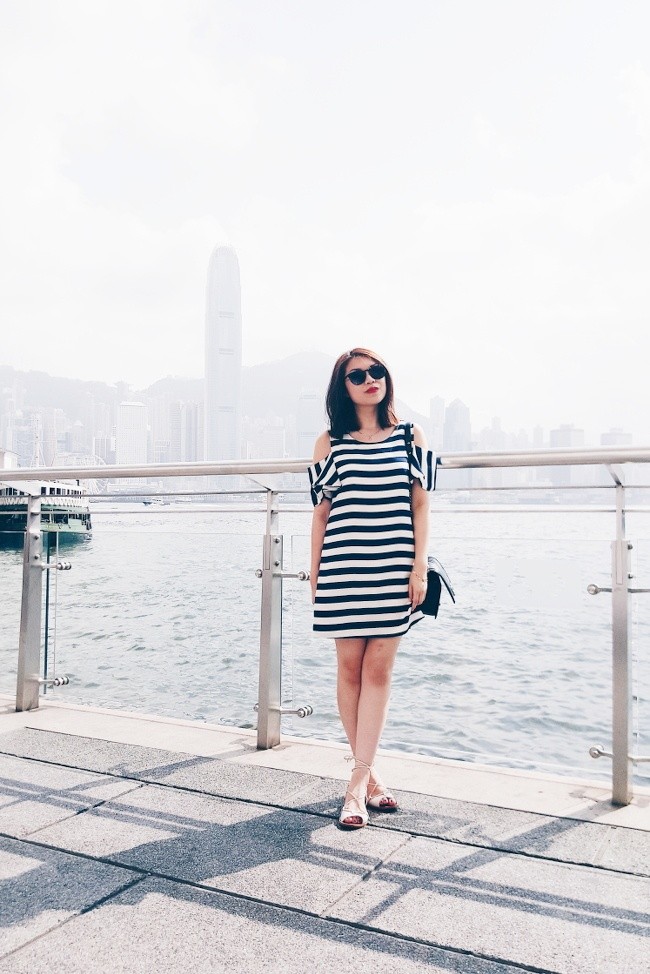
[358,428,381,440]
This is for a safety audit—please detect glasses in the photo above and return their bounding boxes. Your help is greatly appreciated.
[345,363,387,385]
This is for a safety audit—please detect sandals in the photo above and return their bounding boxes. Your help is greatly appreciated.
[339,759,374,827]
[366,779,399,812]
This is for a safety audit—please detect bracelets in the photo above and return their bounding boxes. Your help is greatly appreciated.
[412,561,429,583]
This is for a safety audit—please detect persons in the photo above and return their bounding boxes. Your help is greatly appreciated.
[307,349,455,828]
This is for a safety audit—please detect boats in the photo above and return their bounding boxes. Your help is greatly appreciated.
[0,432,92,550]
[142,498,164,507]
[175,498,192,505]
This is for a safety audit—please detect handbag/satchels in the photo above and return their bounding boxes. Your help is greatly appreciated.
[419,556,456,618]
